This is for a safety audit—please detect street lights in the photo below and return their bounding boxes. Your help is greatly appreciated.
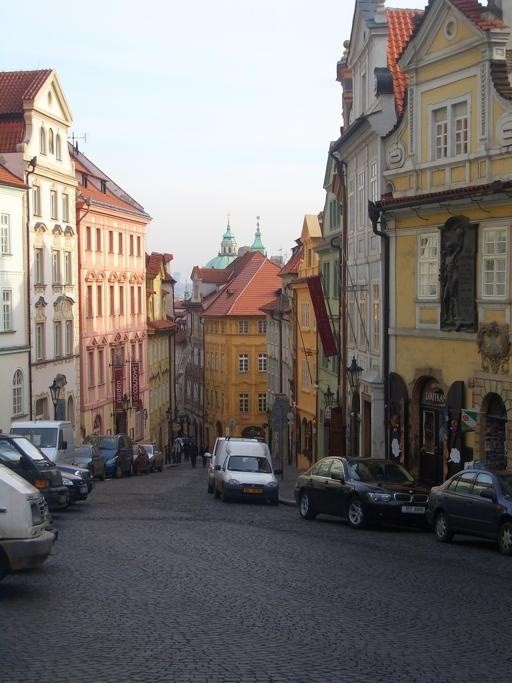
[324,385,336,456]
[346,356,364,457]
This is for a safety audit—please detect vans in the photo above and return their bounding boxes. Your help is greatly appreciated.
[203,437,282,506]
[83,433,134,479]
[0,461,58,580]
[0,429,70,510]
[9,420,74,462]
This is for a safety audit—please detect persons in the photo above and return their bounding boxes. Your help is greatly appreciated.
[180,440,210,467]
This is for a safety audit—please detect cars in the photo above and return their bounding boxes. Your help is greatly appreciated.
[74,445,106,481]
[134,444,163,474]
[0,446,93,501]
[295,454,430,529]
[427,468,512,555]
